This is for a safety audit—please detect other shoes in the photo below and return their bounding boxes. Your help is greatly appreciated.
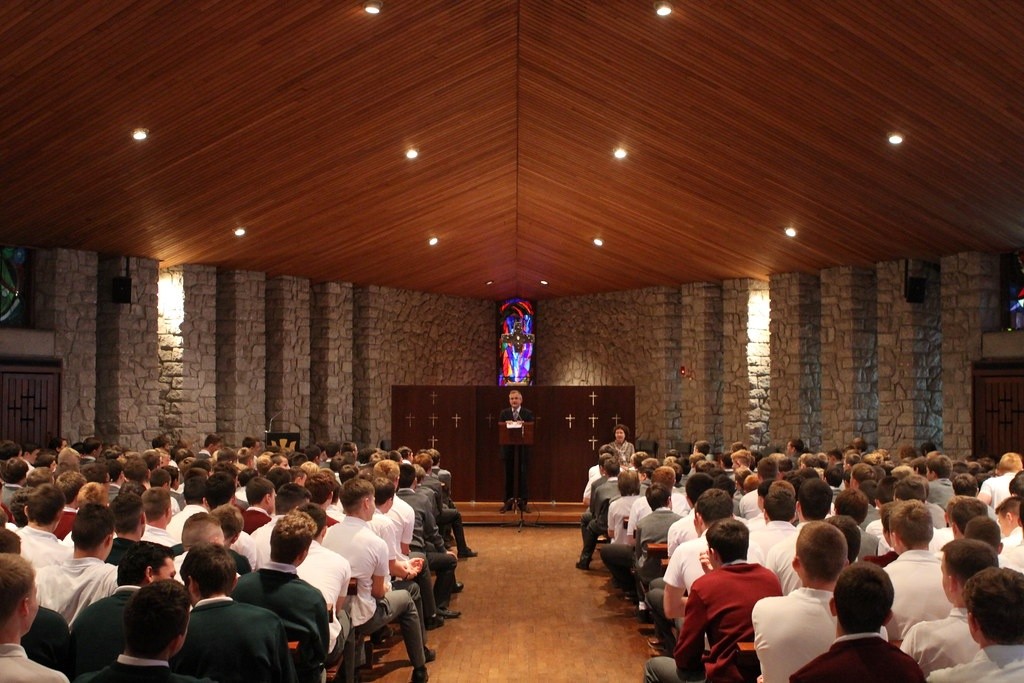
[412,669,428,683]
[500,502,512,514]
[518,503,531,513]
[425,613,443,630]
[637,609,655,624]
[424,644,435,662]
[457,549,478,558]
[372,626,393,645]
[440,608,460,617]
[647,636,675,653]
[444,537,457,548]
[451,582,464,593]
[576,560,589,571]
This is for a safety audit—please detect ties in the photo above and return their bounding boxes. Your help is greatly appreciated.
[514,409,518,420]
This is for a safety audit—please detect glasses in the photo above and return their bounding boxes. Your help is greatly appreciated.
[105,479,113,484]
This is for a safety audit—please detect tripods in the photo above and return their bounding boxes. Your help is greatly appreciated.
[500,408,544,533]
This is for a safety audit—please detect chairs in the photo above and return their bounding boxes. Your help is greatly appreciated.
[634,440,656,458]
[327,604,348,683]
[621,518,760,683]
[671,439,693,456]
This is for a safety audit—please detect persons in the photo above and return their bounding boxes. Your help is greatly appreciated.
[927,567,1024,683]
[70,543,176,675]
[170,541,293,683]
[755,489,796,575]
[0,552,73,683]
[0,435,478,660]
[643,518,783,683]
[880,499,955,642]
[74,579,209,683]
[321,477,431,682]
[234,511,329,669]
[751,519,889,683]
[663,488,780,652]
[107,491,146,588]
[0,526,71,682]
[609,424,636,467]
[768,479,834,570]
[576,438,1024,648]
[900,539,1000,674]
[788,562,929,683]
[498,390,536,513]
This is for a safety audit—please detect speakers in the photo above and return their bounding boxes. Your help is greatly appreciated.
[111,276,131,304]
[905,276,926,303]
